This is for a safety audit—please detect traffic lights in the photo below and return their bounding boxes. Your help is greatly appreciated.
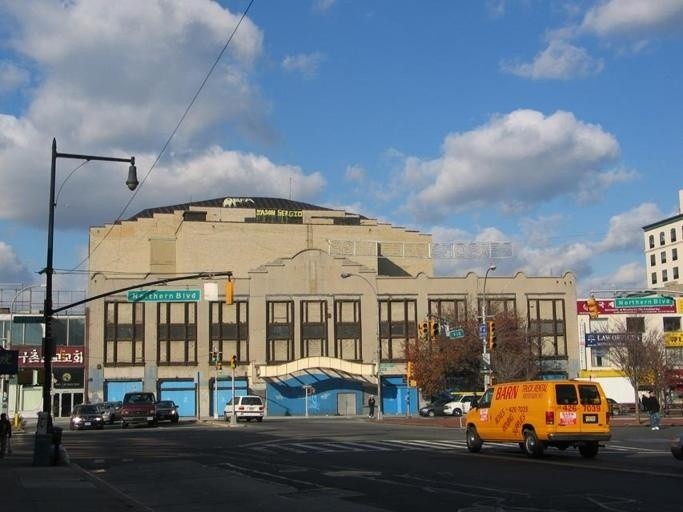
[429,317,440,341]
[416,321,430,344]
[488,320,498,353]
[229,354,237,372]
[210,350,223,373]
[586,296,599,319]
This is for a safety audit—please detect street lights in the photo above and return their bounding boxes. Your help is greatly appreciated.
[482,263,497,392]
[35,137,139,465]
[341,272,383,422]
[3,278,48,428]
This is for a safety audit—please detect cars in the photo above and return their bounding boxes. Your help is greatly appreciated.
[607,398,625,417]
[419,393,485,416]
[222,394,265,423]
[69,400,123,430]
[155,400,180,423]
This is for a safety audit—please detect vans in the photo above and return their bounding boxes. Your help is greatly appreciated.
[463,381,612,459]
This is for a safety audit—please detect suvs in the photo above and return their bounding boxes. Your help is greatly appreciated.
[121,392,158,429]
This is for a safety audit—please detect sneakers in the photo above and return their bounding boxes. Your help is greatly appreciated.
[368,416,375,419]
[651,427,660,431]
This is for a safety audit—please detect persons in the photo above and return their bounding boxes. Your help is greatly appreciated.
[0,413,12,458]
[647,390,659,430]
[642,394,649,411]
[368,394,376,418]
